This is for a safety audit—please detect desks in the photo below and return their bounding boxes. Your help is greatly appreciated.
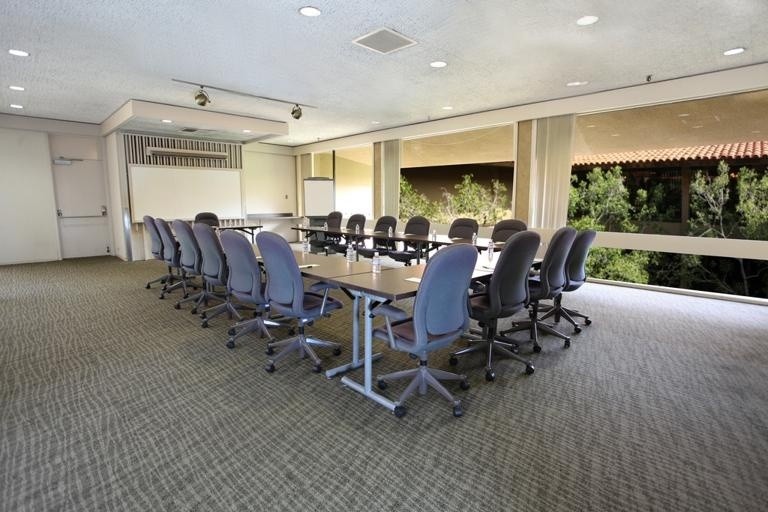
[171,222,265,244]
[238,241,394,379]
[328,249,597,415]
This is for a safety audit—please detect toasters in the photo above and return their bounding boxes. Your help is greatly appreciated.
[488,239,495,259]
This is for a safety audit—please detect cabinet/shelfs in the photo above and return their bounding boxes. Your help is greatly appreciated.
[290,223,493,265]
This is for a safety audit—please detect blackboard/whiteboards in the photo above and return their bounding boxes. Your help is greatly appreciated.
[52,159,107,216]
[304,177,334,219]
[127,163,243,223]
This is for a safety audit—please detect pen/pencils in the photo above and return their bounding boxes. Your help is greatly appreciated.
[301,266,312,268]
[482,266,489,269]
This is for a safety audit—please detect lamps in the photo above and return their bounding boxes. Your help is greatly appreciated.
[172,79,318,120]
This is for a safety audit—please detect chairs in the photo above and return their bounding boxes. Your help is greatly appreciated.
[144,215,180,293]
[447,228,542,381]
[491,219,528,242]
[172,219,228,313]
[191,223,256,329]
[256,231,345,374]
[387,215,430,268]
[154,218,202,304]
[448,218,479,239]
[540,227,598,334]
[499,227,579,355]
[377,243,480,416]
[304,211,344,257]
[324,213,367,258]
[353,216,398,263]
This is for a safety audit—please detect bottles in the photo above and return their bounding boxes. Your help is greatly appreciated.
[303,237,309,256]
[356,224,360,233]
[323,222,329,232]
[346,243,354,262]
[372,251,381,274]
[472,232,478,245]
[432,230,437,241]
[388,226,393,238]
